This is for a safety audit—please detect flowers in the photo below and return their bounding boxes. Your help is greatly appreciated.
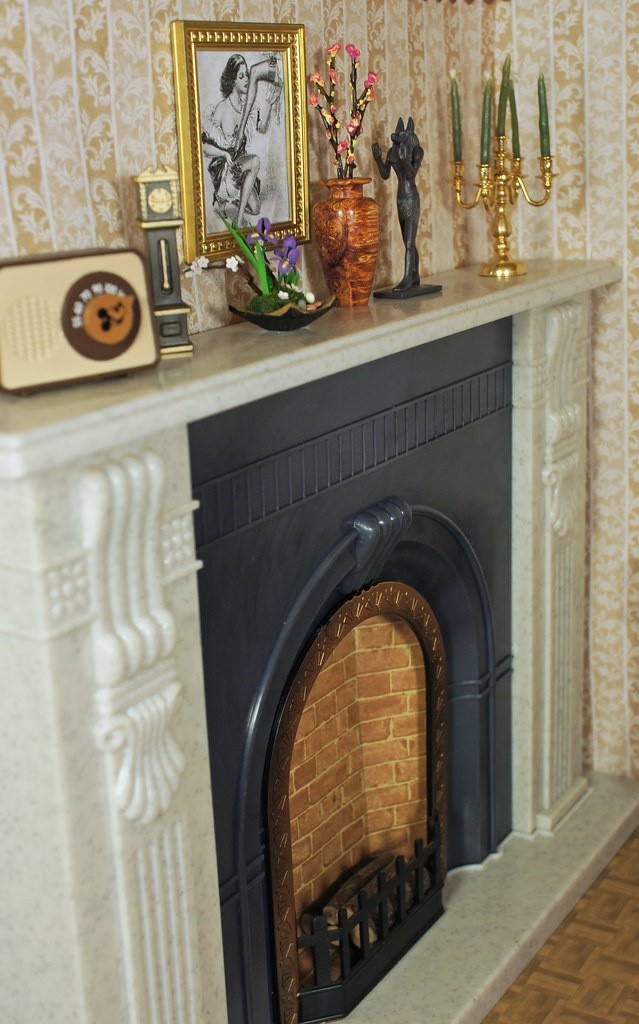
[215,210,317,314]
[302,41,378,178]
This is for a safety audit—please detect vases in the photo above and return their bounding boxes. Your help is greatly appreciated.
[314,176,382,308]
[227,292,337,331]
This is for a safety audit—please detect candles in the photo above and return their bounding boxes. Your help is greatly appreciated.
[510,81,520,159]
[537,74,551,156]
[482,78,491,163]
[452,70,462,162]
[498,55,512,135]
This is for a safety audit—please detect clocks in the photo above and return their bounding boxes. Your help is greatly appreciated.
[131,163,193,353]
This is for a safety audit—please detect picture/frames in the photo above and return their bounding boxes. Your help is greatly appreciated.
[170,19,312,263]
